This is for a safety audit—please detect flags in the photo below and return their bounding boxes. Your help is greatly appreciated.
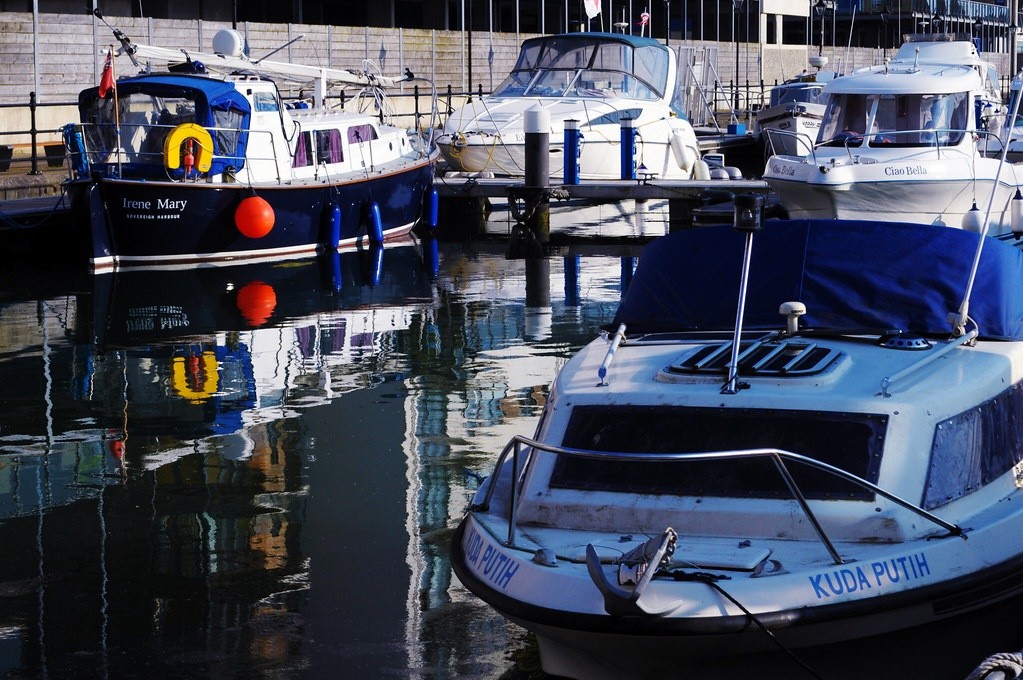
[583,0,601,18]
[98,49,114,98]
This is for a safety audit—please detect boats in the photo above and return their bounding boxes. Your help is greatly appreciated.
[450,194,1023,653]
[60,62,440,276]
[977,75,1023,153]
[431,33,702,186]
[753,57,834,157]
[762,61,1023,244]
